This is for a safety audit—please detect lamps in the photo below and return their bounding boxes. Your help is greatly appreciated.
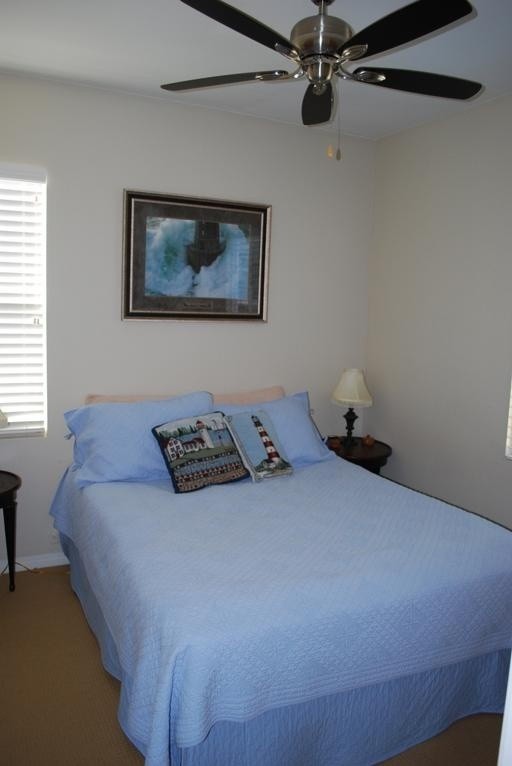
[332,369,373,447]
[291,56,354,96]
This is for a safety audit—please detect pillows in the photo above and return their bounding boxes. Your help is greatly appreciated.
[63,387,338,494]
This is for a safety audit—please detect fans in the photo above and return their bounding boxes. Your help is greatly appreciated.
[161,0,482,125]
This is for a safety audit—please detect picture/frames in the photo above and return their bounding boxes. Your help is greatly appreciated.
[121,187,272,323]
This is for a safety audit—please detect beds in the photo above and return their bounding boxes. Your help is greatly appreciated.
[48,453,512,766]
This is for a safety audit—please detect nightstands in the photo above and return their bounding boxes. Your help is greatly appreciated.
[323,436,392,476]
[0,469,22,592]
